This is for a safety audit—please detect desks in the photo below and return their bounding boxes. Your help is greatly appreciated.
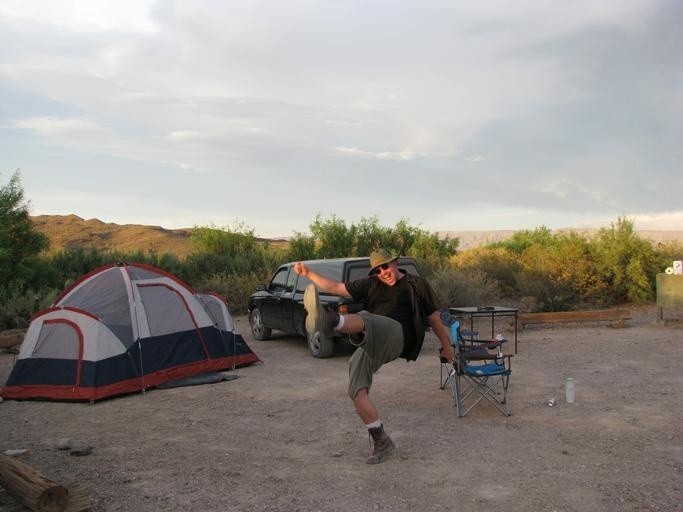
[447,305,519,353]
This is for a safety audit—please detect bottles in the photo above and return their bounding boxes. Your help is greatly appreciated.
[565,377,575,403]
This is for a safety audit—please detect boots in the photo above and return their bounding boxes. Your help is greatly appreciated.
[303,282,341,335]
[366,424,395,466]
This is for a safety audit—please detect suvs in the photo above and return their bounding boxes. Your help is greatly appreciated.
[246,256,426,359]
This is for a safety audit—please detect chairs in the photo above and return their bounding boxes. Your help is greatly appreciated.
[439,319,514,422]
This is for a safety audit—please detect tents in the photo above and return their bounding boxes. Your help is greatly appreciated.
[0,259,267,402]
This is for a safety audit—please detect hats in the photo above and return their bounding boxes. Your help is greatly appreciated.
[365,248,398,276]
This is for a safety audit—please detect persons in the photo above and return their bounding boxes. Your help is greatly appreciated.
[293,247,453,466]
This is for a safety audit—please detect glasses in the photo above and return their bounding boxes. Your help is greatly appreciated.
[374,264,388,275]
